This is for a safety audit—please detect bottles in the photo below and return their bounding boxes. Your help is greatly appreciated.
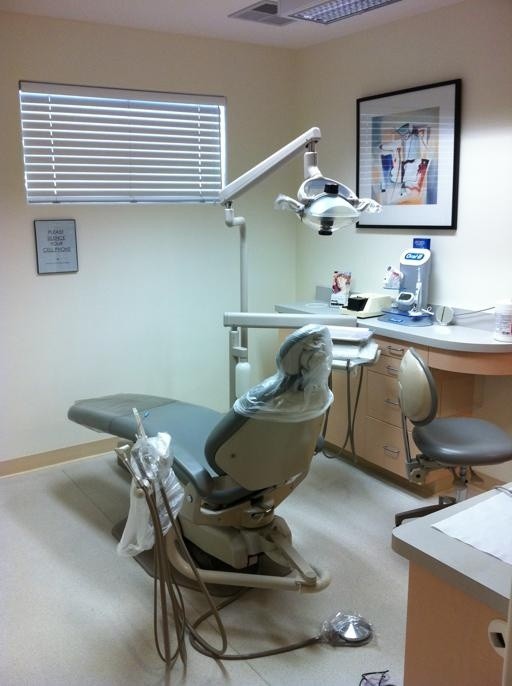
[493,302,512,344]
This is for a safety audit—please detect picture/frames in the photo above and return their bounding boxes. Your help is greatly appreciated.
[356,79,461,230]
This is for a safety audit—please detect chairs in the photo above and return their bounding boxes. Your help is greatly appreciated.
[67,326,334,598]
[396,351,510,524]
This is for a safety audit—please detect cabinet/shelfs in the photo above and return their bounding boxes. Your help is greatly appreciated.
[277,314,474,497]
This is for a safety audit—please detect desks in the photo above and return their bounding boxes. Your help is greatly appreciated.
[391,484,509,686]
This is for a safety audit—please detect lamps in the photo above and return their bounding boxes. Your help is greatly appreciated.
[219,125,382,411]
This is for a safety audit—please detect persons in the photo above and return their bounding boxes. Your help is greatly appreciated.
[331,274,350,304]
